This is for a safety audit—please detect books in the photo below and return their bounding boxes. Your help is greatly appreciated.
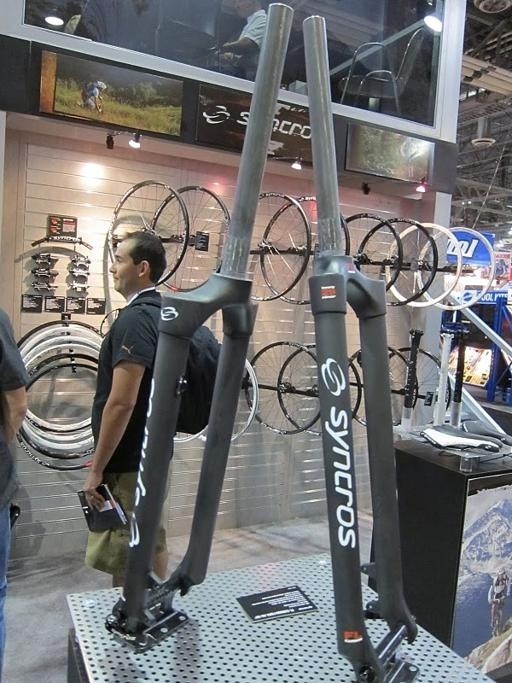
[76,483,129,532]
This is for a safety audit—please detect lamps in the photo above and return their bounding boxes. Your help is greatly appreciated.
[423,9,443,31]
[103,127,147,150]
[360,175,428,194]
[45,8,65,25]
[269,155,305,169]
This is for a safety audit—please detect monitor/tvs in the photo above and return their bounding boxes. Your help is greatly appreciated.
[449,345,492,386]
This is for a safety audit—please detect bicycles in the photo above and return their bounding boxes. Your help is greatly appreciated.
[74,97,105,115]
[488,594,509,637]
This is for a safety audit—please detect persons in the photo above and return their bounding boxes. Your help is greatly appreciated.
[1,303,30,681]
[82,228,172,585]
[80,80,108,113]
[206,0,268,83]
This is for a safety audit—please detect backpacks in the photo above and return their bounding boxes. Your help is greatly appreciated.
[131,297,220,434]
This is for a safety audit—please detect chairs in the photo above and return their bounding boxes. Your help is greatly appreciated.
[158,2,222,71]
[337,27,424,117]
